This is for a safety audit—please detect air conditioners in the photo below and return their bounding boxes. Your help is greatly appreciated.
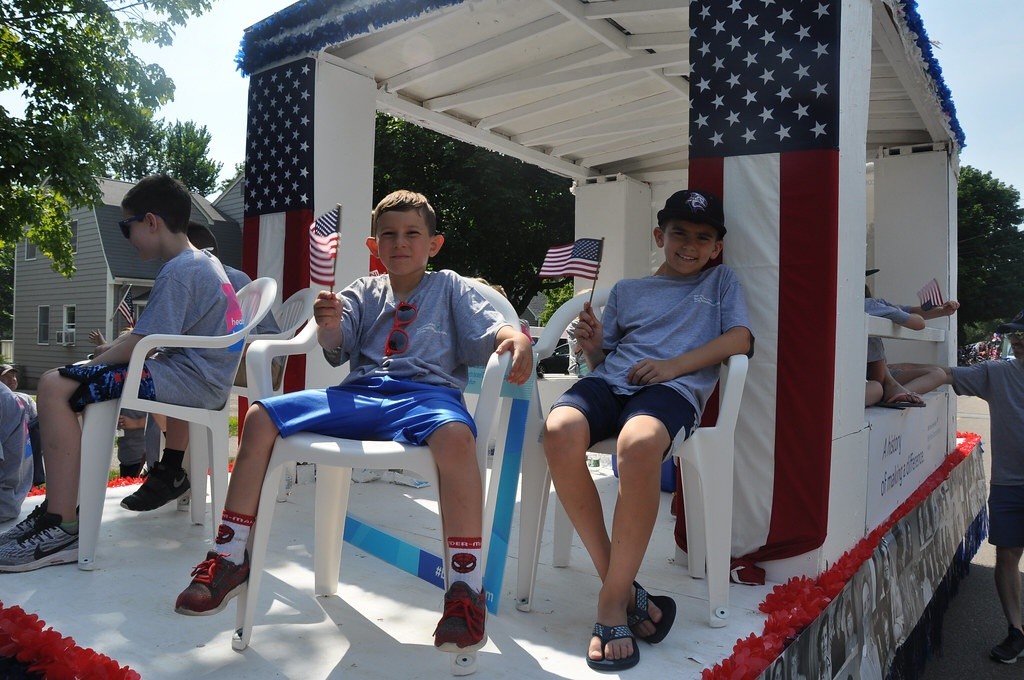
[56,331,75,344]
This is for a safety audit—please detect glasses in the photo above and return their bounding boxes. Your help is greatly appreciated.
[1006,328,1024,340]
[384,301,417,356]
[118,215,144,239]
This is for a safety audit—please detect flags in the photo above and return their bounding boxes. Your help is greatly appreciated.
[540,236,604,280]
[309,202,342,287]
[118,283,136,328]
[916,278,944,312]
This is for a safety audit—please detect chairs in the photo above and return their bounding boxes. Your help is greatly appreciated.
[78,276,750,651]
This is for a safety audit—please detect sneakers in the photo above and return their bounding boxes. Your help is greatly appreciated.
[173,548,249,617]
[0,499,79,572]
[434,580,488,653]
[120,461,191,513]
[991,625,1024,664]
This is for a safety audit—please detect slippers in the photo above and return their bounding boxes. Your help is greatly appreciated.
[587,621,641,673]
[627,579,677,644]
[875,391,926,407]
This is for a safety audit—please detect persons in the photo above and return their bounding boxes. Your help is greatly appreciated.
[988,332,1001,360]
[888,310,1024,663]
[175,190,534,655]
[0,175,245,572]
[864,267,961,407]
[543,191,756,671]
[0,221,284,523]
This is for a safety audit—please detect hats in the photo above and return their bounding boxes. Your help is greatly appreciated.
[657,189,727,239]
[0,363,20,377]
[997,310,1024,334]
